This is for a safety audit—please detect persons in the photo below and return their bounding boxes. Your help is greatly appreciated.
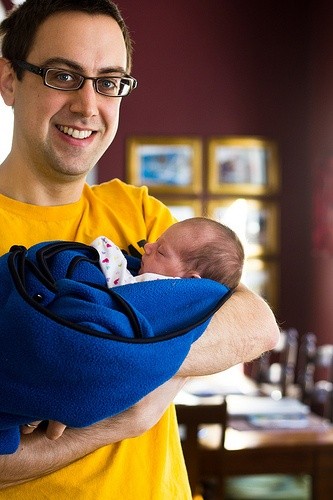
[0,0,278,500]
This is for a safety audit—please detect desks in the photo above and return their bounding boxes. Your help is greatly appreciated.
[174,389,333,500]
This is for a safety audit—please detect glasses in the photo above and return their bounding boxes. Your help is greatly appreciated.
[19,61,137,97]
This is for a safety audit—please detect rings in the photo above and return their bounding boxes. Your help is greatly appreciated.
[25,423,38,429]
